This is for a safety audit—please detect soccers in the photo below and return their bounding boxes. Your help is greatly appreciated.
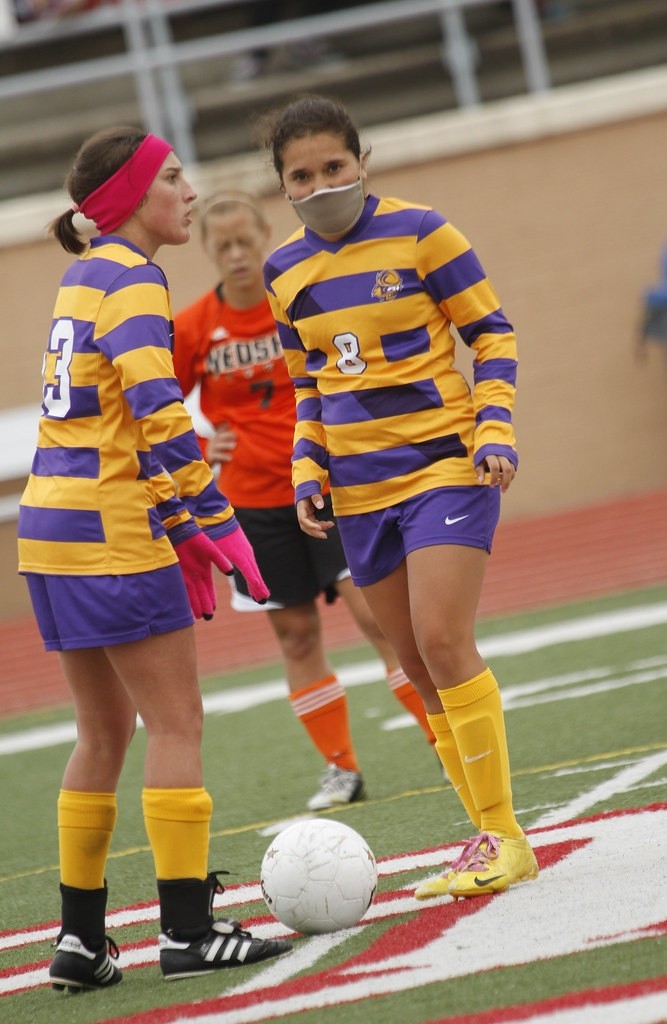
[259,818,380,935]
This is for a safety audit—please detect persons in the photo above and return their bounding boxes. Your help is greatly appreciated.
[17,128,294,994]
[264,95,538,899]
[169,188,450,811]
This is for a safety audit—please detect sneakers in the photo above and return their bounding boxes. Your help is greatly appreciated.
[415,835,481,900]
[307,763,367,810]
[449,832,539,900]
[158,918,294,981]
[49,931,123,993]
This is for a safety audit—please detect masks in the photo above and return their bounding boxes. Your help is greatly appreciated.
[284,159,364,236]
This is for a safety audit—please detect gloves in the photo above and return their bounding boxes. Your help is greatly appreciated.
[172,530,235,622]
[213,524,272,608]
